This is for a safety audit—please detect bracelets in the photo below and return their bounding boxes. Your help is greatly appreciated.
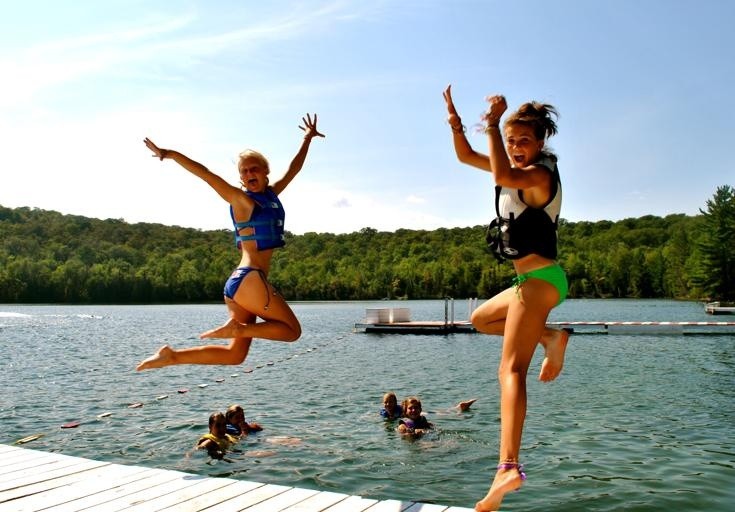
[485,123,501,134]
[451,123,466,136]
[303,133,312,141]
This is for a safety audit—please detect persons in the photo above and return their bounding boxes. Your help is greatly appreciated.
[444,84,568,512]
[380,392,478,420]
[185,411,276,460]
[134,112,326,372]
[398,398,433,437]
[225,406,298,445]
